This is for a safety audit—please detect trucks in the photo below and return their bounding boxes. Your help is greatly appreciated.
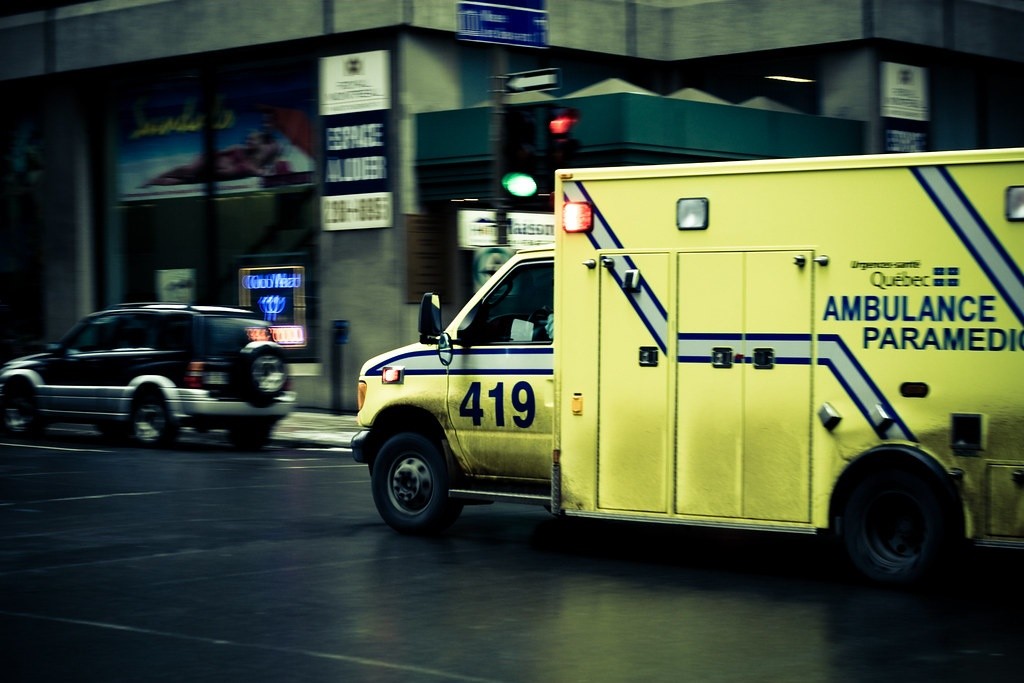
[350,146,1024,588]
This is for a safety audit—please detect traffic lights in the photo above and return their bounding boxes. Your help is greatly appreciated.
[501,104,539,212]
[542,103,583,192]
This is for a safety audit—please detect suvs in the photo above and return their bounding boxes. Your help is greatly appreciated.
[0,301,298,454]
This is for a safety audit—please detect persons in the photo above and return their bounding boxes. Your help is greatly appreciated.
[136,129,278,189]
